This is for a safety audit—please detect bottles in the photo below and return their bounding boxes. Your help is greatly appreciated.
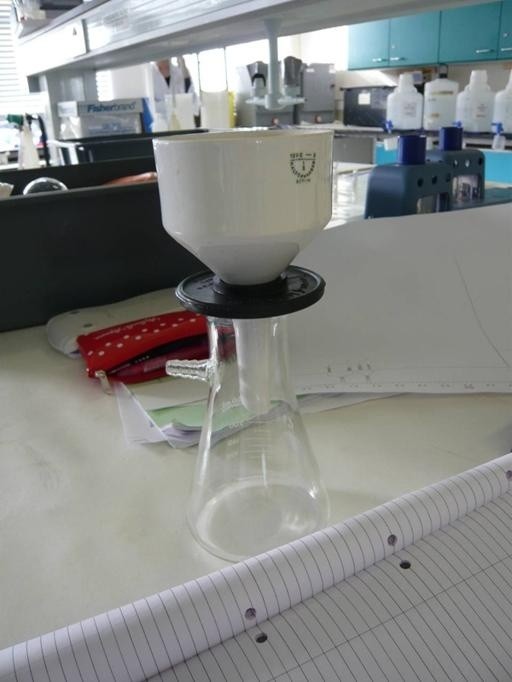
[384,74,424,131]
[493,71,512,134]
[456,69,497,130]
[148,123,334,564]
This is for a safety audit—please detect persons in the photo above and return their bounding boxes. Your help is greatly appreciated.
[149,55,195,113]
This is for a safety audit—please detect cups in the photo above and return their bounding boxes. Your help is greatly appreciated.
[166,94,195,130]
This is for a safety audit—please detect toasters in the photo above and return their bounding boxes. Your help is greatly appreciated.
[424,69,458,127]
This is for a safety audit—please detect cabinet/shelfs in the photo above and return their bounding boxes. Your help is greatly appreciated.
[439,0,512,67]
[347,8,440,72]
